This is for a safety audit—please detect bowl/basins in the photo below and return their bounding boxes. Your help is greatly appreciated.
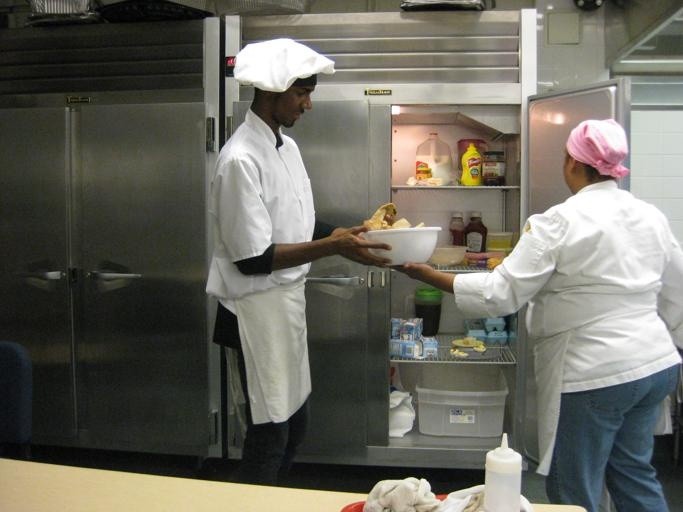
[430,245,469,268]
[359,227,442,266]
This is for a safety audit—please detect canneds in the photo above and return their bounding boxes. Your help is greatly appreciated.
[481,151,506,186]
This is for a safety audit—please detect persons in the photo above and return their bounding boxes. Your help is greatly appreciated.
[206,38,392,488]
[388,119,683,512]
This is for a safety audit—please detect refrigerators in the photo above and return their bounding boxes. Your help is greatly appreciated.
[225,75,633,473]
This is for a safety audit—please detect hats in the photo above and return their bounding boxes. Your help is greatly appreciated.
[232,38,336,94]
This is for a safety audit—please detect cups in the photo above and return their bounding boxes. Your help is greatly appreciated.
[403,287,444,336]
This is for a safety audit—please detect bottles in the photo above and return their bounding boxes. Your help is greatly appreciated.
[484,432,523,512]
[465,211,487,265]
[449,212,466,245]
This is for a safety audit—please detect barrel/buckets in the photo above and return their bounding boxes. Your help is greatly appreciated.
[415,132,453,185]
